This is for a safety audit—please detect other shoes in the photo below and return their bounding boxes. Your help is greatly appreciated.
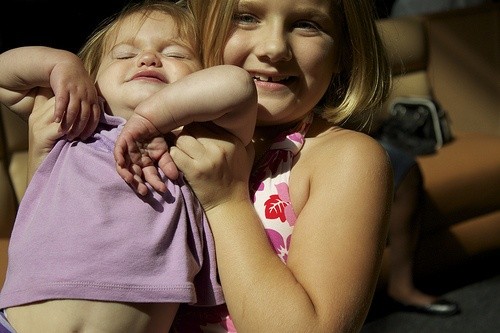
[389,291,458,316]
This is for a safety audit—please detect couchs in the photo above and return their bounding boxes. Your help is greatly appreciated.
[363,10,500,272]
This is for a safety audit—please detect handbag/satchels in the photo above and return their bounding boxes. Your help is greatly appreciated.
[383,97,453,155]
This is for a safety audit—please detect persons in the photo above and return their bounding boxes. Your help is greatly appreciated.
[0,2,258,332]
[348,128,462,317]
[25,0,391,333]
